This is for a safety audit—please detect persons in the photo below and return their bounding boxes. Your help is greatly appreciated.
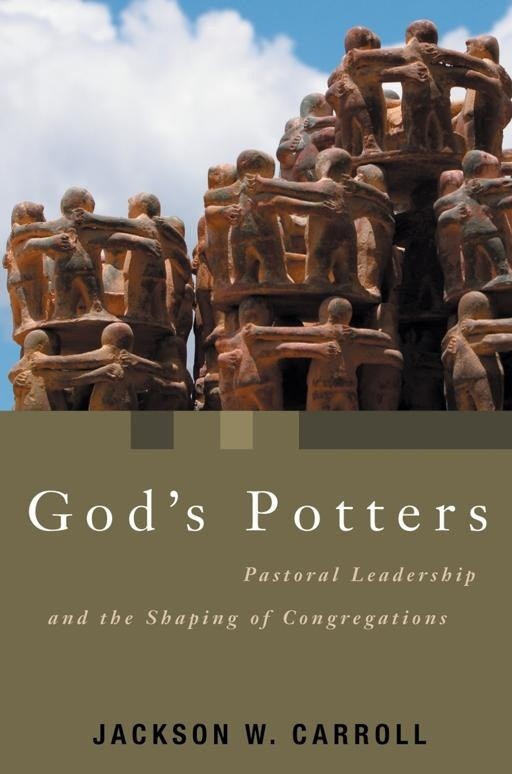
[2,17,510,411]
